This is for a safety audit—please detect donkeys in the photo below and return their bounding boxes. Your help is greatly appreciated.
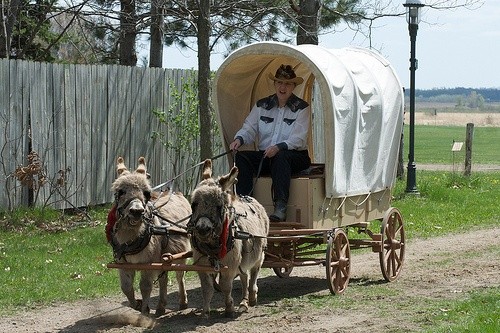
[108,156,193,317]
[189,159,270,319]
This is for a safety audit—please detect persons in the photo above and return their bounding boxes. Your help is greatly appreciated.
[229,64,312,221]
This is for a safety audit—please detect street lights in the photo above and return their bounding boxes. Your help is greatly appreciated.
[402,0,425,195]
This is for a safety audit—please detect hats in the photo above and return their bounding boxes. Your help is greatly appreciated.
[268,64,304,84]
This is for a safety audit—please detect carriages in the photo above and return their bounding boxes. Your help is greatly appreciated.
[103,41,406,312]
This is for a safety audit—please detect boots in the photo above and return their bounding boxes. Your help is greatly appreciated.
[269,200,287,221]
